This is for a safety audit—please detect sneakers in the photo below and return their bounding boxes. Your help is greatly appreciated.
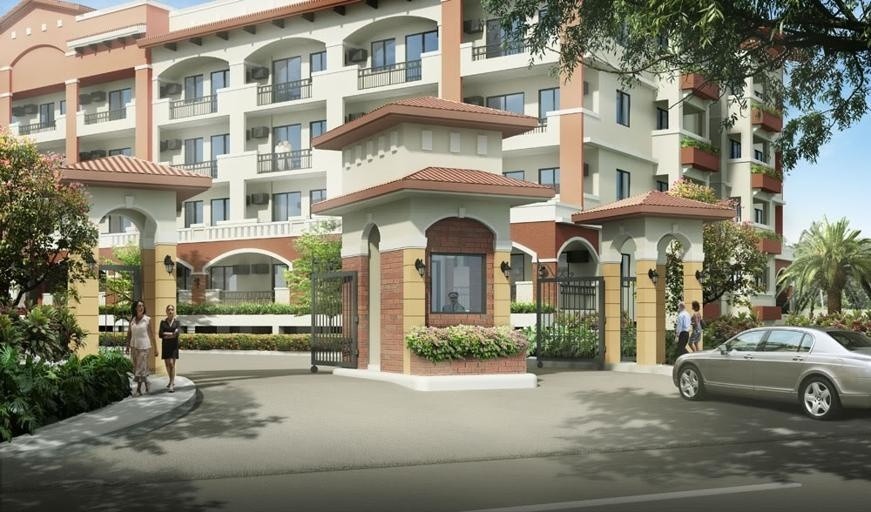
[137,380,175,396]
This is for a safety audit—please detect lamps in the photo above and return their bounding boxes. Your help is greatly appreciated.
[539,264,545,277]
[696,270,706,284]
[647,269,659,285]
[415,257,426,277]
[500,260,512,279]
[165,255,174,273]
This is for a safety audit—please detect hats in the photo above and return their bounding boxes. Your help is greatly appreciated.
[448,292,458,299]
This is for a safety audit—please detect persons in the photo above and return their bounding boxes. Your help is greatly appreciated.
[444,291,466,312]
[690,301,703,352]
[158,305,181,392]
[126,300,158,397]
[676,302,693,354]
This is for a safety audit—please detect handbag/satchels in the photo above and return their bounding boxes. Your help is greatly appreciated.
[700,319,707,328]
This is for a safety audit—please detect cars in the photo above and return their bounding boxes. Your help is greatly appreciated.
[673,324,871,421]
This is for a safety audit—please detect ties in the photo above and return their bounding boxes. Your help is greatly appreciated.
[452,304,454,312]
